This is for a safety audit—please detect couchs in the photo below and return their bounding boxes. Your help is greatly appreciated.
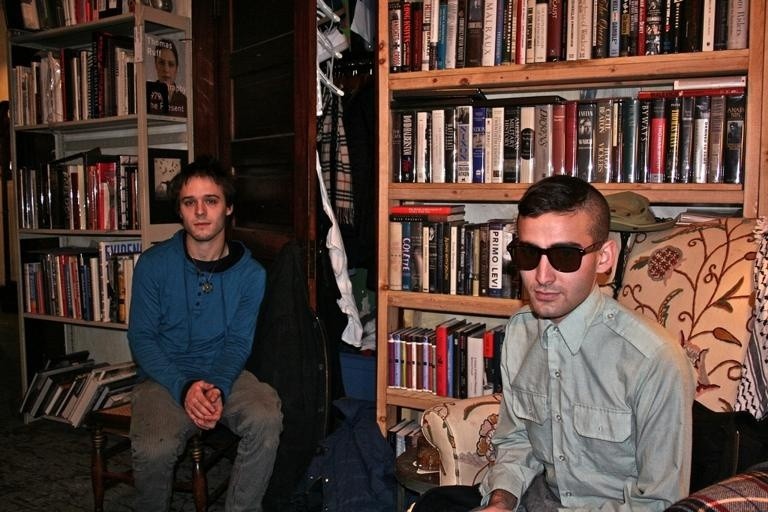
[419,218,768,512]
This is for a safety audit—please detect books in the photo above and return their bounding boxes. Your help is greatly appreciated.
[13,31,136,126]
[387,315,505,399]
[22,239,141,325]
[388,201,524,300]
[393,76,749,185]
[387,1,748,75]
[19,150,140,231]
[18,350,136,429]
[673,209,739,225]
[7,0,172,35]
[385,416,423,457]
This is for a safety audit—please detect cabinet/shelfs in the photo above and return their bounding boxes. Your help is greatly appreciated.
[0,0,193,426]
[378,0,768,437]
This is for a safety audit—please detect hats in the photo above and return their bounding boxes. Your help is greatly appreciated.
[603,192,675,232]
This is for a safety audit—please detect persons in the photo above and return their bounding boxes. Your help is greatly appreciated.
[148,39,188,118]
[130,159,282,512]
[413,178,694,512]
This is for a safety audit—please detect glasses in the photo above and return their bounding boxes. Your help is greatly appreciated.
[507,237,602,272]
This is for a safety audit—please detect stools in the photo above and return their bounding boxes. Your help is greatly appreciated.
[87,400,241,512]
